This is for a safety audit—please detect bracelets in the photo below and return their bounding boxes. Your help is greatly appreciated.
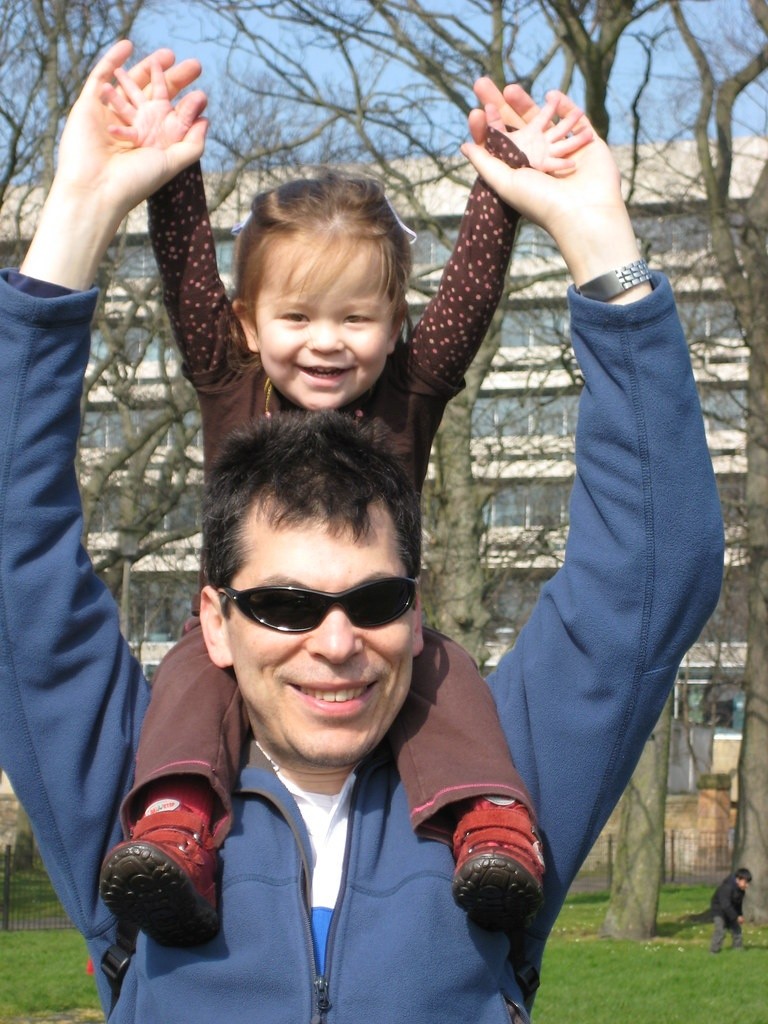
[574,259,654,302]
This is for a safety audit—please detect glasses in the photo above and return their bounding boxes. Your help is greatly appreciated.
[225,576,418,633]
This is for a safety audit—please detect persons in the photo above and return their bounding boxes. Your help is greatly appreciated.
[0,28,728,1023]
[709,866,752,953]
[101,52,595,950]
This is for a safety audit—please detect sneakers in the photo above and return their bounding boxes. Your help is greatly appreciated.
[98,798,220,949]
[452,795,546,931]
